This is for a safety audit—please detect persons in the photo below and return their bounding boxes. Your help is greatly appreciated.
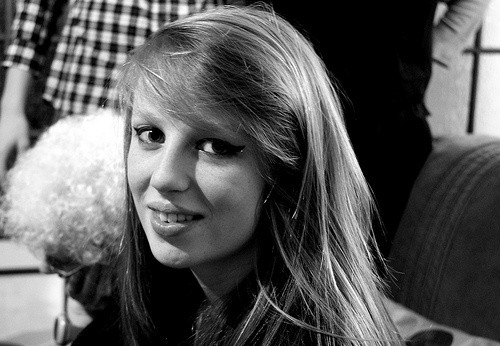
[246,0,490,275]
[0,0,241,190]
[71,8,412,346]
[0,109,126,319]
